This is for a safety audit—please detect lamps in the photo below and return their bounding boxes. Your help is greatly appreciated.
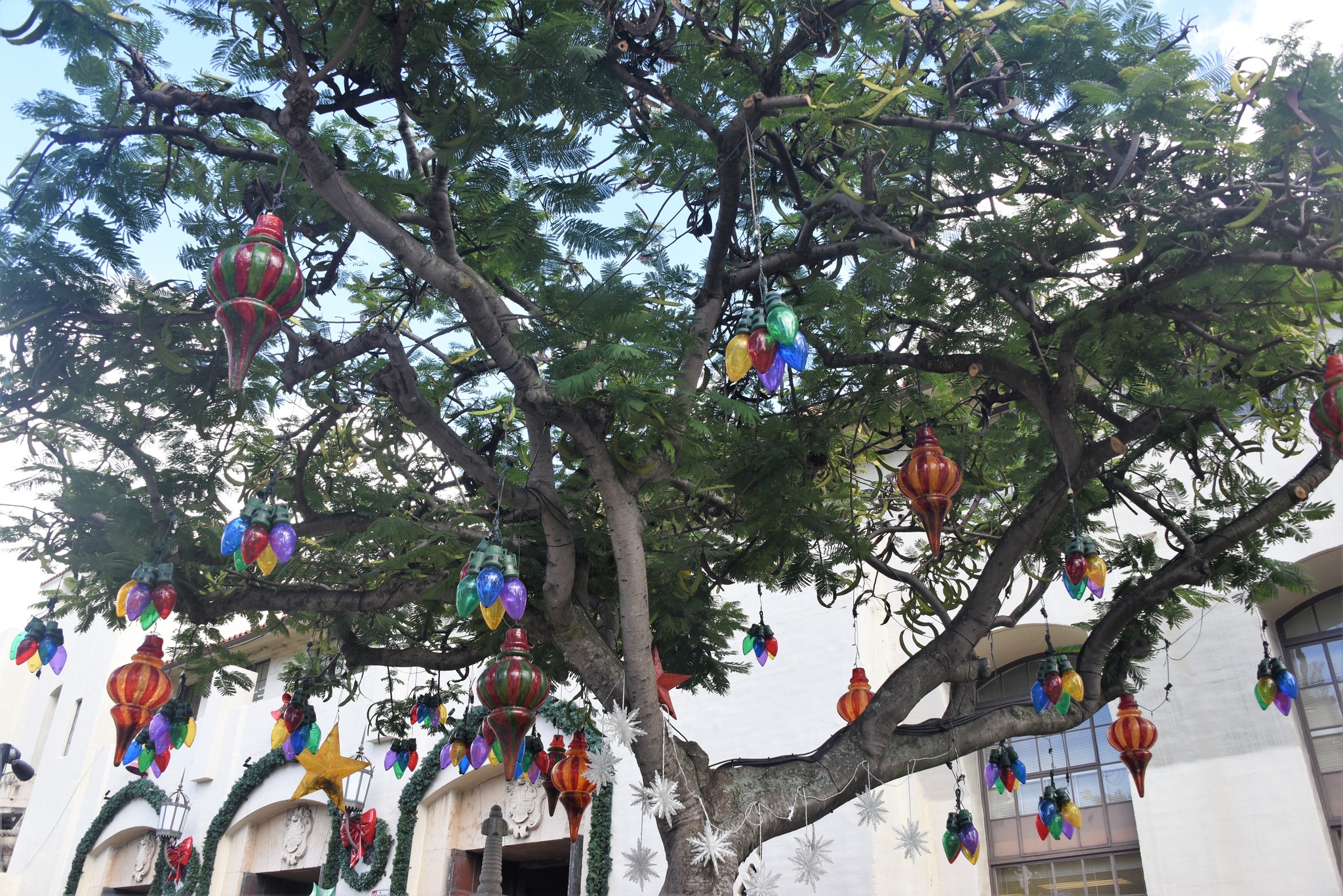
[456,533,527,631]
[1031,654,1084,716]
[895,427,963,556]
[836,668,876,726]
[384,738,419,779]
[438,628,550,785]
[943,809,980,865]
[1254,657,1299,717]
[1035,786,1082,840]
[221,496,297,576]
[580,698,933,896]
[651,645,692,720]
[984,746,1027,795]
[1106,692,1158,798]
[1062,536,1107,601]
[106,635,174,766]
[550,731,600,843]
[9,615,68,675]
[122,698,197,779]
[410,693,447,728]
[539,734,568,817]
[205,212,307,394]
[116,560,178,630]
[724,289,809,396]
[1309,353,1343,461]
[270,689,321,761]
[290,722,372,814]
[742,623,778,667]
